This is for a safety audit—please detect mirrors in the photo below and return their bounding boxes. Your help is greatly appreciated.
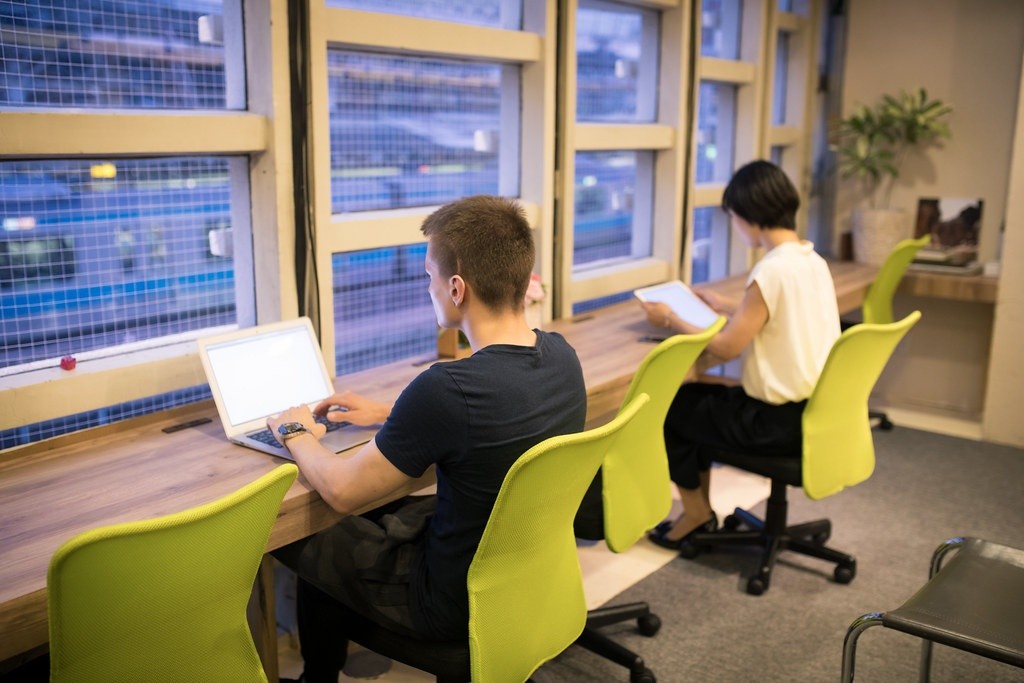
[911,195,984,267]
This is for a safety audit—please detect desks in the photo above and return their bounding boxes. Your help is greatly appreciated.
[0,253,999,683]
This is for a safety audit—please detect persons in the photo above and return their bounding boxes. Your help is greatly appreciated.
[265,194,587,683]
[649,158,845,551]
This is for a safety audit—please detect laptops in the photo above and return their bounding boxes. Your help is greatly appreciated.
[909,198,984,267]
[198,316,386,462]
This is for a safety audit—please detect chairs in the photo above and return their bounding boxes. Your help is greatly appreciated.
[840,536,1024,683]
[840,232,935,431]
[677,308,923,594]
[570,315,729,683]
[348,392,651,683]
[48,460,301,683]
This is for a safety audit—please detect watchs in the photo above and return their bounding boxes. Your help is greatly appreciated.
[278,422,313,447]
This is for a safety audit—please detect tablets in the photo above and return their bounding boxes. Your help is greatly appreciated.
[634,280,720,329]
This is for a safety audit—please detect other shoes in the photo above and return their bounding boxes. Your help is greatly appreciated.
[649,511,717,559]
[279,673,305,683]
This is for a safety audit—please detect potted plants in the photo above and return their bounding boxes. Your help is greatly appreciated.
[825,87,955,267]
